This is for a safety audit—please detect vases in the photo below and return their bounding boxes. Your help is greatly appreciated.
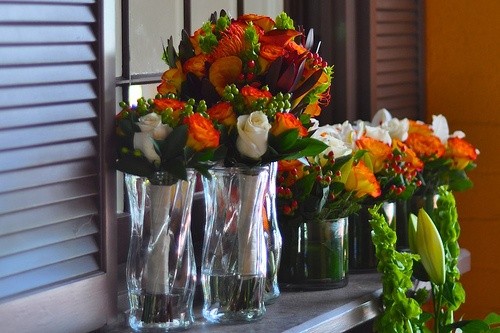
[263,162,283,306]
[199,160,268,324]
[124,167,195,332]
[402,197,449,272]
[275,215,352,292]
[349,199,397,276]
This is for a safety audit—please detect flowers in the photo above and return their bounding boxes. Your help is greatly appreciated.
[275,110,482,215]
[416,209,446,329]
[106,7,336,184]
